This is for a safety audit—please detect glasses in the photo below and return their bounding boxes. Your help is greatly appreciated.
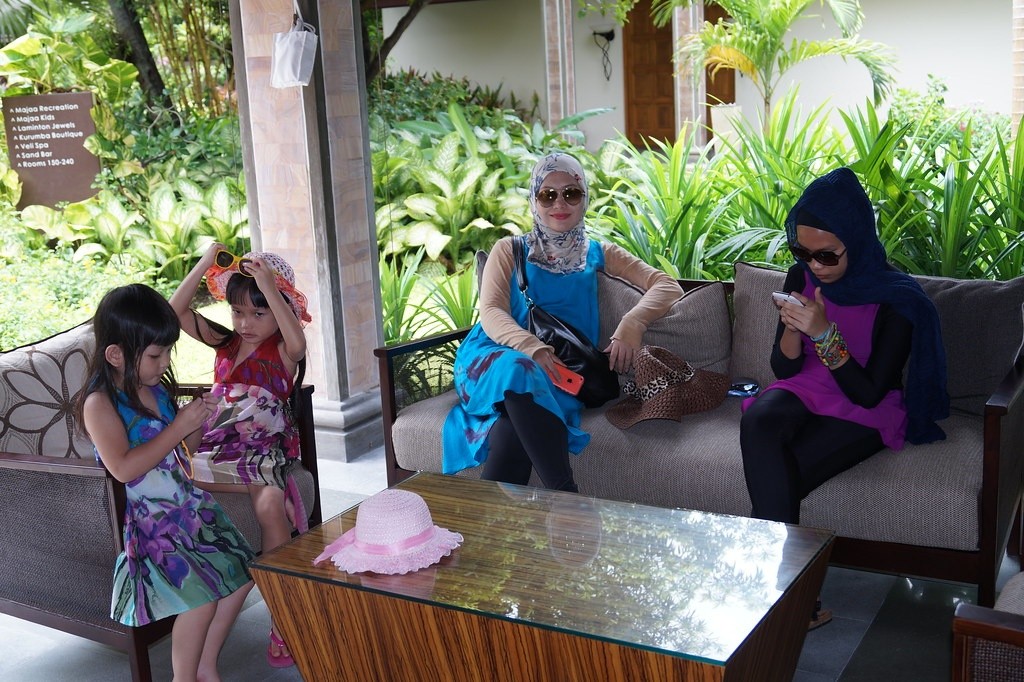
[214,249,283,277]
[789,235,847,266]
[536,187,586,208]
[552,535,596,550]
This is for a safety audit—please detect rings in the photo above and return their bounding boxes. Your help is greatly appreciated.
[611,340,614,344]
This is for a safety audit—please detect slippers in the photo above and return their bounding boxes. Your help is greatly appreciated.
[807,610,832,629]
[269,628,294,668]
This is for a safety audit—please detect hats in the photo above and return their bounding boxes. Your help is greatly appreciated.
[606,344,729,429]
[315,487,464,575]
[205,250,311,329]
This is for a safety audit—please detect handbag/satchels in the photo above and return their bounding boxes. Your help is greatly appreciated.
[270,19,317,88]
[528,304,620,408]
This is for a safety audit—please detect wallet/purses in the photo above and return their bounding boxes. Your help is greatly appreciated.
[728,378,759,398]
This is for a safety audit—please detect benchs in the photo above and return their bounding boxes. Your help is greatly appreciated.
[371,249,1024,601]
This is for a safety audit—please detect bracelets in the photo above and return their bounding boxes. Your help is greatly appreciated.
[811,322,848,366]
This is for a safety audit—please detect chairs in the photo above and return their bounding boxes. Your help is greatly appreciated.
[951,567,1024,682]
[0,308,323,682]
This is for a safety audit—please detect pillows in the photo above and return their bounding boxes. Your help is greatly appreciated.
[597,271,731,392]
[732,260,788,376]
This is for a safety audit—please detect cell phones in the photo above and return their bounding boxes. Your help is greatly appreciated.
[772,290,805,308]
[545,362,584,396]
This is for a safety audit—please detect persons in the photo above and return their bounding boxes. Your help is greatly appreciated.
[75,284,257,682]
[168,243,313,666]
[496,482,602,569]
[740,168,950,633]
[441,153,685,494]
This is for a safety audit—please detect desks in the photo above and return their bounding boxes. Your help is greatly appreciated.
[244,470,838,682]
